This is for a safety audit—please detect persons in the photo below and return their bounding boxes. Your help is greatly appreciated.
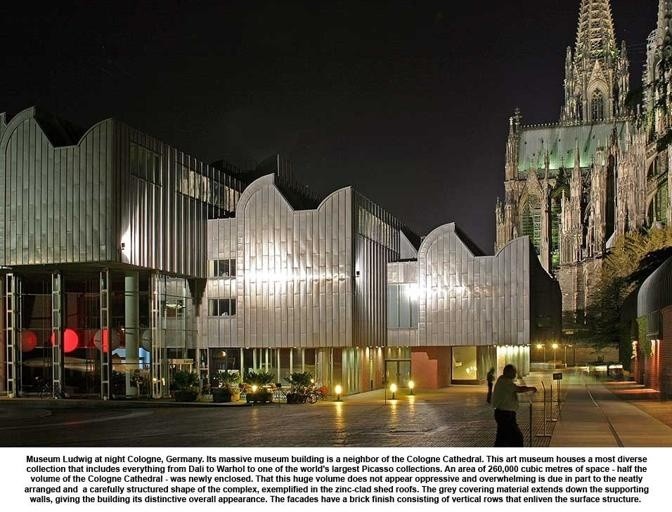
[485,365,497,404]
[490,362,539,448]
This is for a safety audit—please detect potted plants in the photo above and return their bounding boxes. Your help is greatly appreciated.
[169,364,314,404]
